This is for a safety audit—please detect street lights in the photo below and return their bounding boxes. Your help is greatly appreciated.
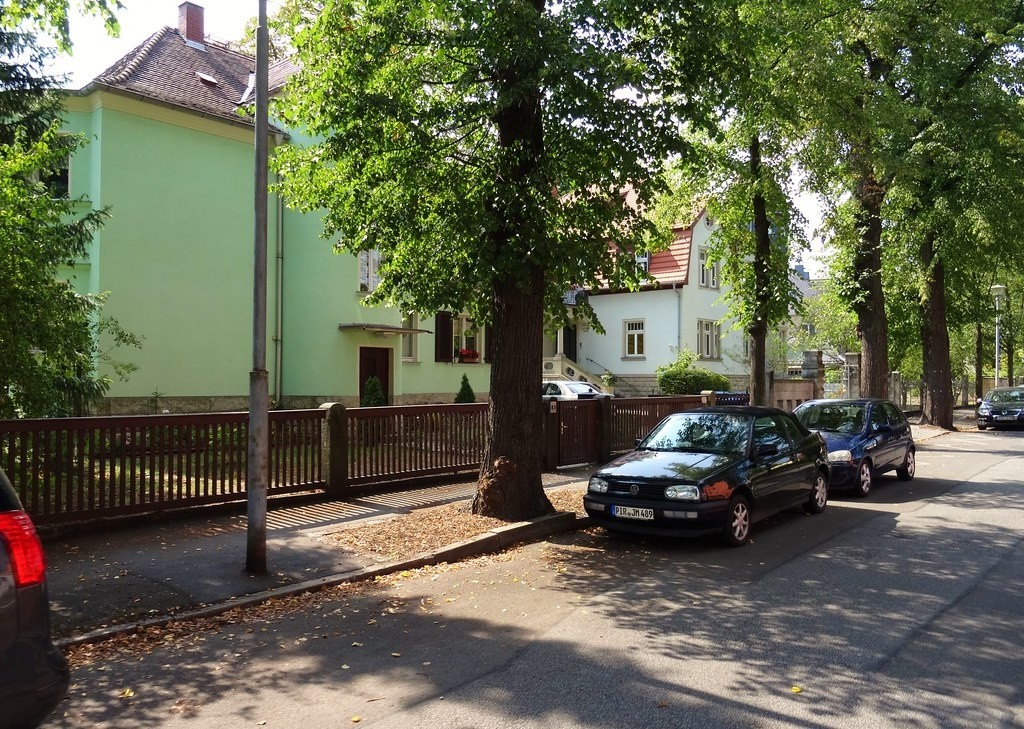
[989,284,1006,390]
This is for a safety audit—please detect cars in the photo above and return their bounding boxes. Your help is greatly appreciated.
[976,387,1024,429]
[786,397,917,497]
[583,403,834,550]
[541,380,617,415]
